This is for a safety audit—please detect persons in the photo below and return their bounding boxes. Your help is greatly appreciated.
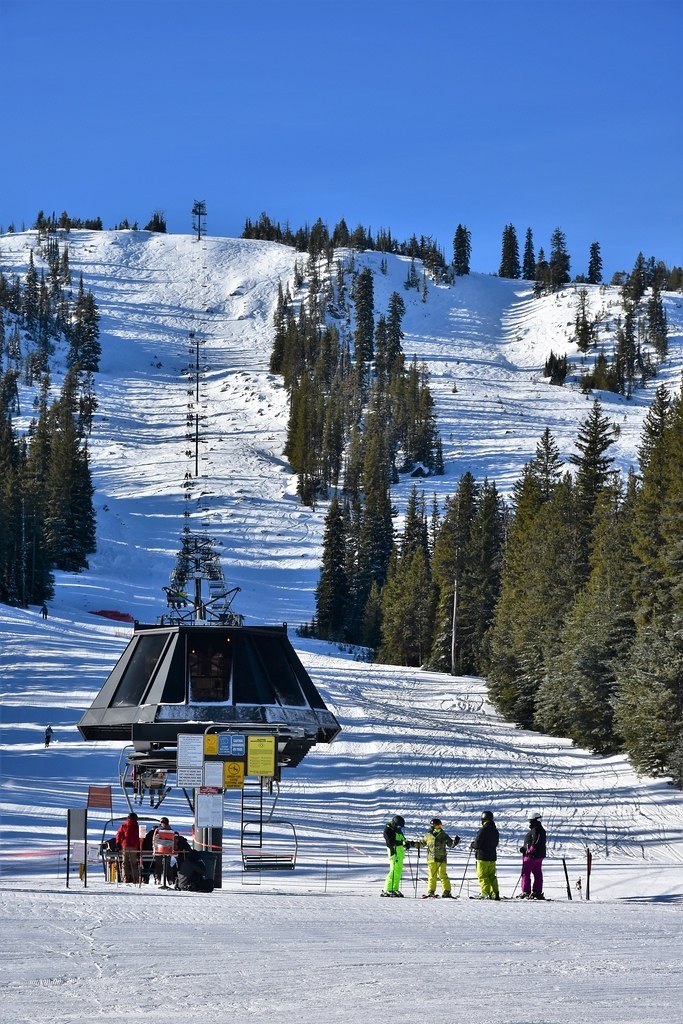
[44,725,54,748]
[469,811,499,900]
[39,604,48,619]
[380,815,460,899]
[130,768,165,807]
[116,813,214,893]
[516,813,546,900]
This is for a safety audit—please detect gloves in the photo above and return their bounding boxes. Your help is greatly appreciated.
[410,841,415,847]
[520,847,526,853]
[405,842,410,850]
[471,841,475,848]
[455,835,460,843]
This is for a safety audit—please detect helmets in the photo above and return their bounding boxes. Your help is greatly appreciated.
[393,815,405,827]
[527,812,542,819]
[430,819,442,826]
[481,811,493,819]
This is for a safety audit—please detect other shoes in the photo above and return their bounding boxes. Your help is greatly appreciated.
[530,893,542,898]
[380,890,400,897]
[394,890,404,897]
[490,891,501,900]
[476,893,488,900]
[442,890,455,898]
[427,891,434,897]
[516,893,531,899]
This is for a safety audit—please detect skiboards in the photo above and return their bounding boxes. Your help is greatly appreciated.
[382,892,560,901]
